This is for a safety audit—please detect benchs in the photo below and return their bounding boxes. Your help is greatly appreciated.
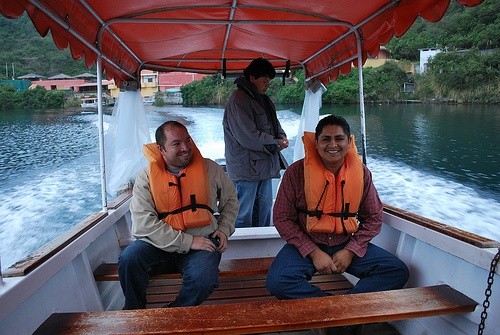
[33,284,479,335]
[220,226,287,257]
[94,257,275,281]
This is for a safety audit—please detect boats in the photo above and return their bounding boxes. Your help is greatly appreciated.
[80,95,100,108]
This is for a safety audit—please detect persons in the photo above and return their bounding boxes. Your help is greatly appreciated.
[264,114,410,335]
[117,121,240,310]
[222,57,289,228]
